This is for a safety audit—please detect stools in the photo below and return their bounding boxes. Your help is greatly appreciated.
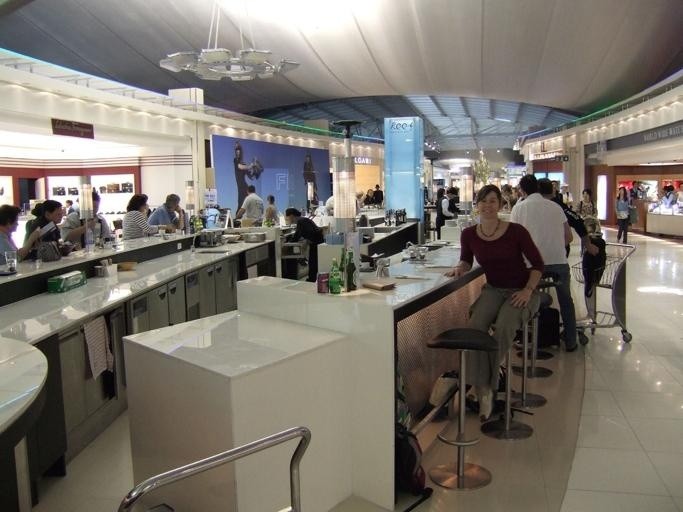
[423,327,502,491]
[478,273,563,442]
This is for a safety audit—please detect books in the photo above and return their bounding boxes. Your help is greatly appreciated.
[32,221,56,243]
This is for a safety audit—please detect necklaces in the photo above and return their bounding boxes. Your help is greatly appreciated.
[478,219,500,239]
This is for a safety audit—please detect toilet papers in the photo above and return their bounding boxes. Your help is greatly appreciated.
[280,217,286,229]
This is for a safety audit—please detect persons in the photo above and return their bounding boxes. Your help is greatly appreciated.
[232,141,255,214]
[284,207,324,282]
[260,194,279,227]
[372,184,383,206]
[0,204,41,273]
[423,181,676,257]
[235,185,265,227]
[440,184,545,425]
[362,189,375,206]
[120,193,172,241]
[300,151,320,214]
[64,200,74,215]
[59,188,111,255]
[581,216,606,299]
[23,199,76,263]
[147,193,184,235]
[509,174,579,355]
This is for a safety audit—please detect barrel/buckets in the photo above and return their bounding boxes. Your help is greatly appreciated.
[204,189,218,208]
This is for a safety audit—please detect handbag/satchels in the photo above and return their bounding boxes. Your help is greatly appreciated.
[580,234,607,299]
[37,239,62,263]
[428,370,459,408]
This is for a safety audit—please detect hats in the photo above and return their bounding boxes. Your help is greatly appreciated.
[284,207,301,217]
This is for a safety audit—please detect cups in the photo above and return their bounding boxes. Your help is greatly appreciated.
[199,231,213,246]
[404,246,428,263]
[4,250,16,272]
[157,211,190,240]
[94,265,112,277]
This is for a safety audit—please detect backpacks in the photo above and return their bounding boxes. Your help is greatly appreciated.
[395,422,427,505]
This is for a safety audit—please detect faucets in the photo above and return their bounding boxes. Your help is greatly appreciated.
[405,241,415,248]
[191,230,203,252]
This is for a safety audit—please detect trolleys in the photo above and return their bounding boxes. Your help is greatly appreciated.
[556,239,636,344]
[117,424,312,511]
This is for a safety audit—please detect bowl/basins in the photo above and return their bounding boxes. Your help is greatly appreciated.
[118,262,136,270]
[223,235,241,243]
[242,232,266,244]
[59,245,76,255]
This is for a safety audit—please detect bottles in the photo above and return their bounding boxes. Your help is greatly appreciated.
[328,246,357,294]
[423,199,437,205]
[263,216,274,227]
[359,214,369,227]
[384,208,406,227]
[189,215,203,235]
[95,232,121,249]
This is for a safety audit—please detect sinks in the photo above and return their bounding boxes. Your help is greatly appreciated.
[197,249,232,254]
[373,226,402,233]
[417,245,443,251]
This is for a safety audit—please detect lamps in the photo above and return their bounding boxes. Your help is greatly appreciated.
[153,0,301,84]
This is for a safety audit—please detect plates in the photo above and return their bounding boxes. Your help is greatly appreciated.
[444,214,470,227]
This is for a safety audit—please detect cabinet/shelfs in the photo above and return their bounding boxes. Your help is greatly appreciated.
[0,230,274,477]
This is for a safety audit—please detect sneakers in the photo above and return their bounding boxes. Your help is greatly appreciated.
[465,385,478,402]
[477,390,495,421]
[565,342,577,353]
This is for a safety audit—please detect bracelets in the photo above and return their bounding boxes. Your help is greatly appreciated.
[524,283,535,293]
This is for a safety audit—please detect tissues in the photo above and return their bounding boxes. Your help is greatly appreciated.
[93,259,118,279]
[47,269,87,294]
